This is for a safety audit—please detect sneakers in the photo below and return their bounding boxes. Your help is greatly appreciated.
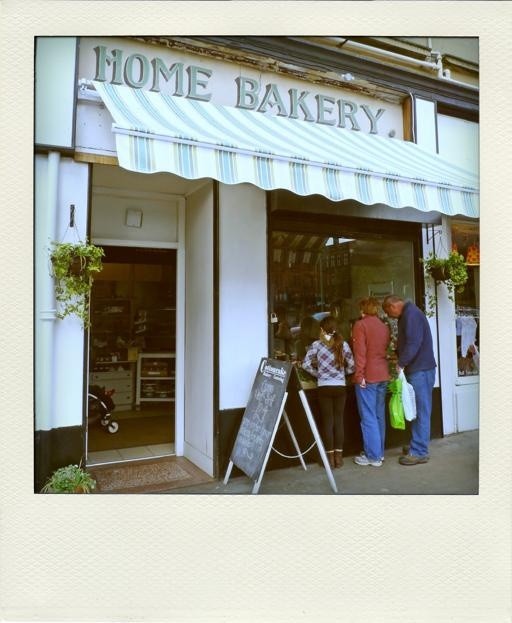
[319,451,344,468]
[400,447,429,465]
[354,451,384,466]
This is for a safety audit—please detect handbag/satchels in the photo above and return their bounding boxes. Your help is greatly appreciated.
[387,368,417,430]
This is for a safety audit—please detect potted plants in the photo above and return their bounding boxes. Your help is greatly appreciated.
[47,240,106,328]
[418,249,468,318]
[40,458,96,494]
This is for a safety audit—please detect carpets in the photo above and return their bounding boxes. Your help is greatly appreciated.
[86,454,215,493]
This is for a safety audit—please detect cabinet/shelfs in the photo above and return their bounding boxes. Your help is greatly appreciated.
[273,270,322,329]
[92,297,134,335]
[136,352,175,411]
[90,360,137,412]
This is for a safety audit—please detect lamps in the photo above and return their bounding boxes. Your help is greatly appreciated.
[278,62,295,72]
[340,72,353,81]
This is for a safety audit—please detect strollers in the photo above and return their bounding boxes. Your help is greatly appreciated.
[88,383,120,434]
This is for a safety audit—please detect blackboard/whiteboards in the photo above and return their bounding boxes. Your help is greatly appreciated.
[230,357,294,482]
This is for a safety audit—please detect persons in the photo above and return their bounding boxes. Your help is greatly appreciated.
[382,295,437,465]
[302,316,355,468]
[352,297,390,467]
